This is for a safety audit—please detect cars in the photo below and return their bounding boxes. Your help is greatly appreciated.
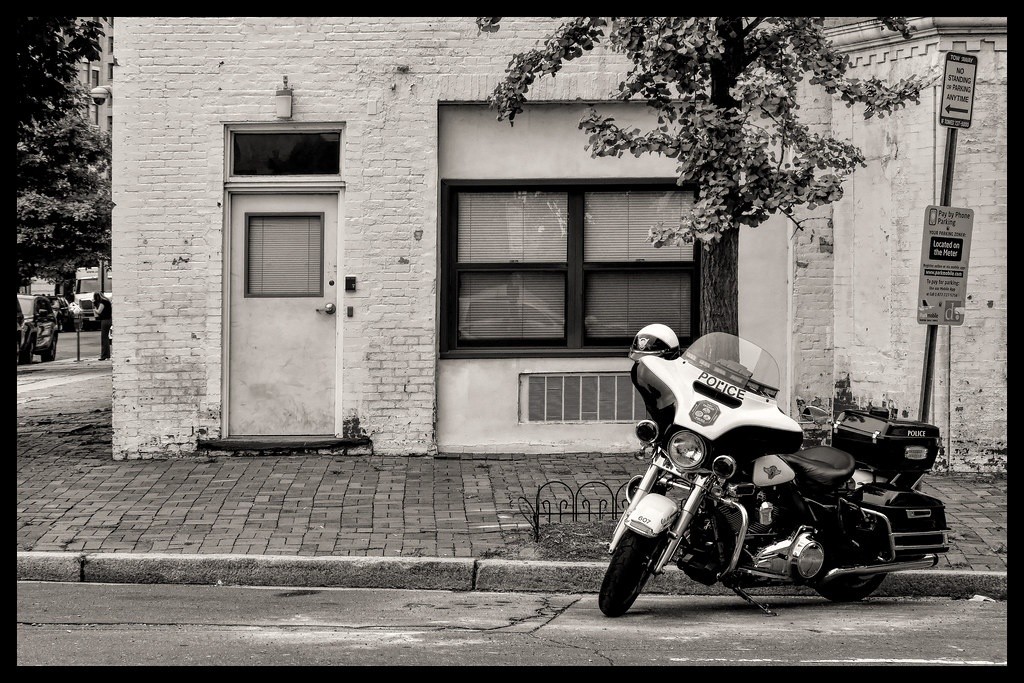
[17,294,59,364]
[34,295,74,334]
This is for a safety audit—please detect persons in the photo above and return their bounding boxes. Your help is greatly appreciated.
[92,292,112,361]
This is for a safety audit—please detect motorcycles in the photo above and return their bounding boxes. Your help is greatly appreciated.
[596,322,953,616]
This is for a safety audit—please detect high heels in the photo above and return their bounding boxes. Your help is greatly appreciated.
[98,356,110,361]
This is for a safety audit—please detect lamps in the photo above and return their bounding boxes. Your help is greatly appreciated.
[275,74,292,118]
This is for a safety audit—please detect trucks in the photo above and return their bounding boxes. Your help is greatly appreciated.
[73,267,112,330]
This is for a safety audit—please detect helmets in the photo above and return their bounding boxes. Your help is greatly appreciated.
[628,323,680,362]
